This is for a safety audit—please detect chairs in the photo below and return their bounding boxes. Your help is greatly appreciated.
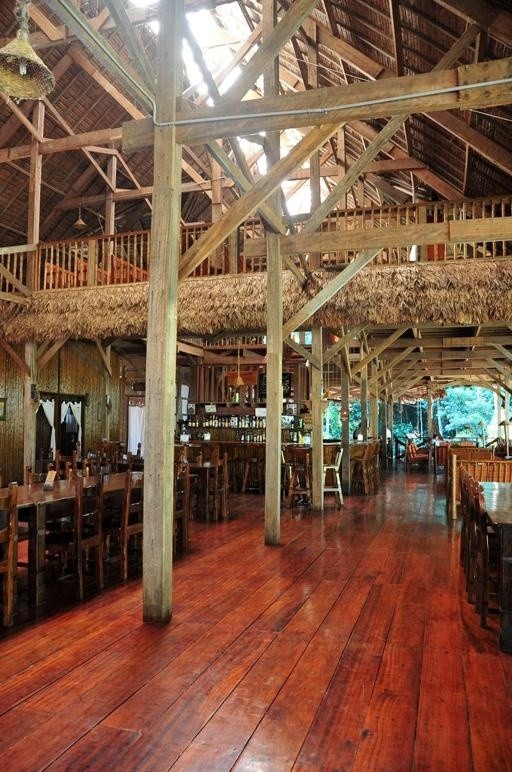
[1,441,236,629]
[235,441,382,509]
[405,440,512,655]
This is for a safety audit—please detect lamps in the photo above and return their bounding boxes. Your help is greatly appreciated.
[0,0,56,100]
[235,441,382,509]
[405,440,512,655]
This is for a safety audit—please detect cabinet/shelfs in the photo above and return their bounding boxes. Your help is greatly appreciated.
[187,414,305,443]
[227,384,255,403]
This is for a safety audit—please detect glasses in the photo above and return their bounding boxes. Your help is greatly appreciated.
[0,0,56,100]
